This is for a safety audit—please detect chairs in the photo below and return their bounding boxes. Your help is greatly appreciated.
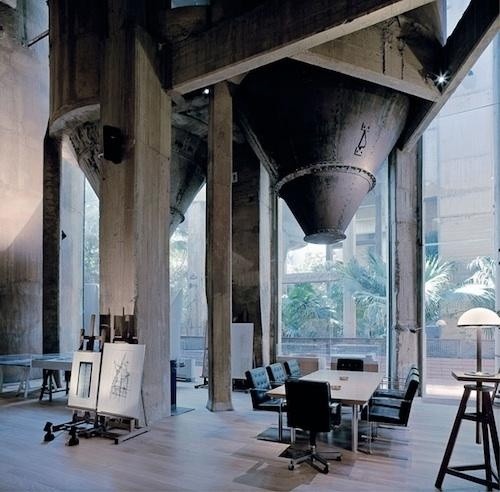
[369,369,421,409]
[268,362,289,385]
[284,377,342,474]
[361,374,420,454]
[245,366,287,443]
[284,358,302,379]
[337,358,364,371]
[375,363,418,398]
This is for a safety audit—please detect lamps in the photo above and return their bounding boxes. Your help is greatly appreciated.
[457,307,500,378]
[437,319,447,328]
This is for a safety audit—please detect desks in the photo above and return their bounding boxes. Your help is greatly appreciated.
[32,354,74,403]
[266,369,386,453]
[0,353,57,397]
[434,370,500,491]
